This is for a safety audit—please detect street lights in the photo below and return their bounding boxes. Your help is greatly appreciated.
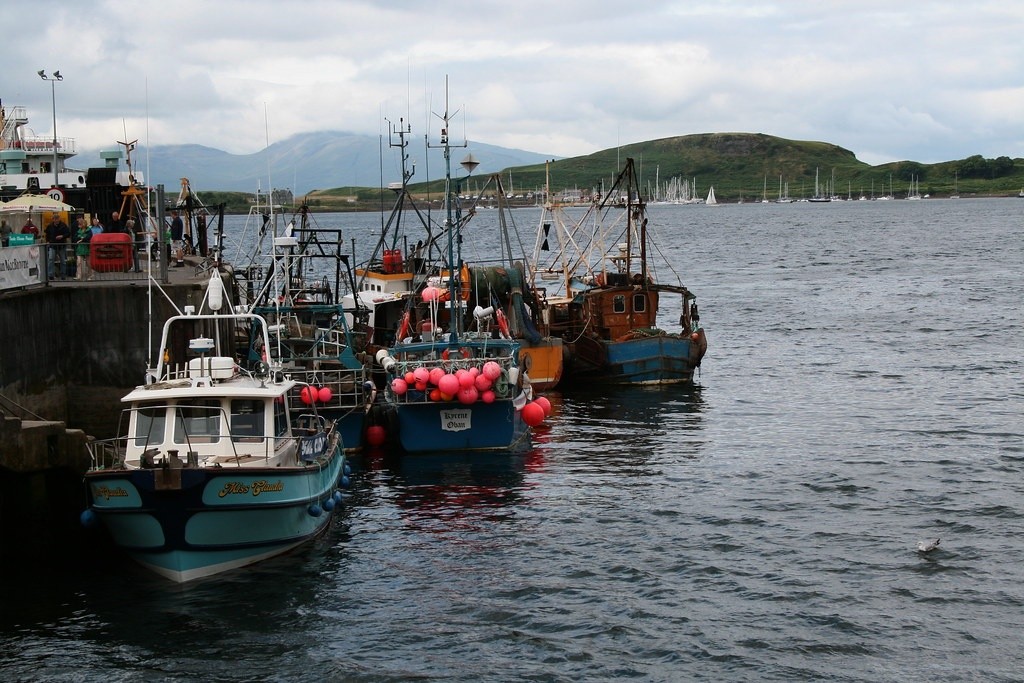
[36,69,63,187]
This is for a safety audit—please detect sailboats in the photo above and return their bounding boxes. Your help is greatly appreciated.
[507,169,513,198]
[489,183,493,199]
[737,165,960,205]
[465,179,471,199]
[516,181,524,200]
[706,186,719,206]
[527,188,533,201]
[473,179,478,199]
[458,183,464,199]
[481,181,486,200]
[535,164,705,208]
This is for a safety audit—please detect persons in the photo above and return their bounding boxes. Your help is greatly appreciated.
[20,218,39,239]
[46,215,70,281]
[0,220,13,247]
[151,211,185,267]
[74,211,143,280]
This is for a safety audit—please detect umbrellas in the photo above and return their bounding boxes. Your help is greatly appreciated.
[0,194,75,225]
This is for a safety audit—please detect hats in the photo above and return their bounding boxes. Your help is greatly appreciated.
[53,214,59,218]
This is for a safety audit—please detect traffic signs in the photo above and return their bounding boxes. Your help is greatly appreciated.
[0,246,43,290]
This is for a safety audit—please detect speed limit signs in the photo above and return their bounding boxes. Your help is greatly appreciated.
[46,187,65,203]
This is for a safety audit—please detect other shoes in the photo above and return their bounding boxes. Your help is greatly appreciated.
[49,277,54,280]
[136,270,143,273]
[87,278,91,280]
[173,262,184,267]
[152,259,157,262]
[73,278,80,281]
[61,278,66,280]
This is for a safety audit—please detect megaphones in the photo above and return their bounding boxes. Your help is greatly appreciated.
[53,71,59,76]
[38,70,44,76]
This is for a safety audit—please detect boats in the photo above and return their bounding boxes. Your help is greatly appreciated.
[166,173,374,337]
[0,95,153,251]
[375,73,555,448]
[554,155,707,388]
[77,224,355,585]
[1018,188,1024,197]
[333,116,615,339]
[240,235,370,455]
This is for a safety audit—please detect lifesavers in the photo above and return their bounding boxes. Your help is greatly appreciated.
[496,309,510,338]
[397,311,410,340]
